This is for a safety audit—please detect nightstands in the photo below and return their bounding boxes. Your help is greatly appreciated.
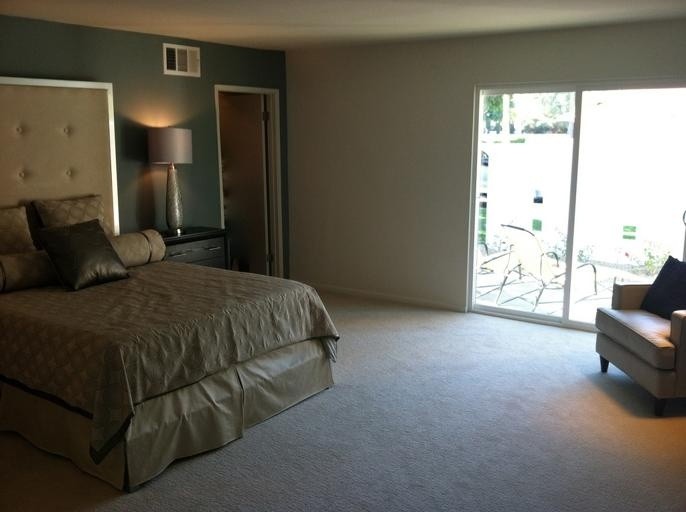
[159,227,232,270]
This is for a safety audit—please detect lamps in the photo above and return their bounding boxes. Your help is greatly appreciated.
[148,127,193,234]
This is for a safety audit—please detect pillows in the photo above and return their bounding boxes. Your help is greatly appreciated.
[640,255,686,320]
[109,229,166,269]
[0,251,53,292]
[40,218,130,292]
[0,206,36,254]
[31,194,105,232]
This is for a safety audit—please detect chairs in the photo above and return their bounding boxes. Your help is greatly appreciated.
[474,223,597,312]
[596,284,686,416]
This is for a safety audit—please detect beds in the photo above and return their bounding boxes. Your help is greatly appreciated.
[0,76,340,494]
